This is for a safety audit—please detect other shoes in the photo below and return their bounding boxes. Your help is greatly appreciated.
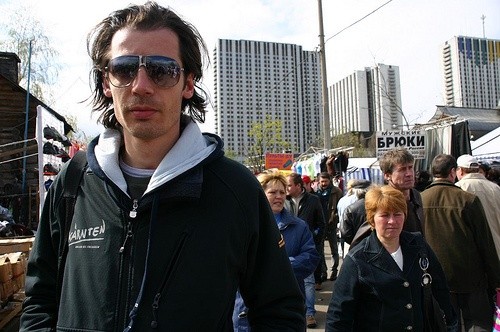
[330,272,337,281]
[315,282,322,290]
[43,126,73,192]
[321,272,327,283]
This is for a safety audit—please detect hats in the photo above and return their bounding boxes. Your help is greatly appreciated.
[356,180,371,188]
[318,172,330,178]
[457,154,479,168]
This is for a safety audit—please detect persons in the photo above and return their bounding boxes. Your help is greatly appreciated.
[349,148,425,250]
[337,178,372,260]
[232,172,320,332]
[454,155,500,260]
[18,0,307,332]
[291,149,349,182]
[301,171,345,291]
[1,183,22,221]
[420,154,500,332]
[414,171,432,192]
[478,164,500,187]
[283,173,326,328]
[325,186,458,332]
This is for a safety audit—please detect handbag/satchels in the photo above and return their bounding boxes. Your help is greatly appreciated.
[423,285,455,332]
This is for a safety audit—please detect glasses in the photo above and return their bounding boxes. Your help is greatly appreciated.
[105,55,185,88]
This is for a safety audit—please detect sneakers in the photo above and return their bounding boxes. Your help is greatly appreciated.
[307,316,317,327]
